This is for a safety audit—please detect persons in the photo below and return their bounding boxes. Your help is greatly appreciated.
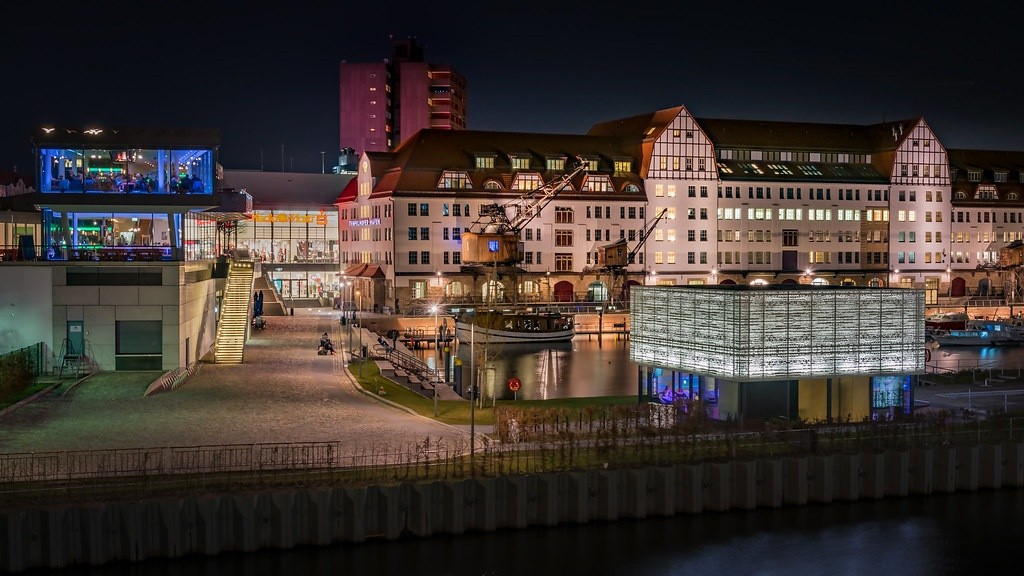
[321,333,336,355]
[378,336,389,346]
[395,299,400,313]
[663,386,672,397]
[58,173,204,193]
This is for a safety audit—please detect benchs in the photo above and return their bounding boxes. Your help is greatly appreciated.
[318,346,328,355]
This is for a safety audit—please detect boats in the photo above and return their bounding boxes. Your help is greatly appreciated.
[450,308,577,345]
[925,302,1024,347]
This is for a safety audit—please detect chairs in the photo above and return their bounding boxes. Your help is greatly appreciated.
[51,179,203,192]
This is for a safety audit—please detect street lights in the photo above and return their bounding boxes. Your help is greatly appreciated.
[320,151,326,174]
[356,290,362,379]
[431,305,438,418]
[281,144,286,172]
[347,282,352,360]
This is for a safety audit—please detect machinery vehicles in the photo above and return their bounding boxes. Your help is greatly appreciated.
[460,152,591,277]
[577,207,669,271]
